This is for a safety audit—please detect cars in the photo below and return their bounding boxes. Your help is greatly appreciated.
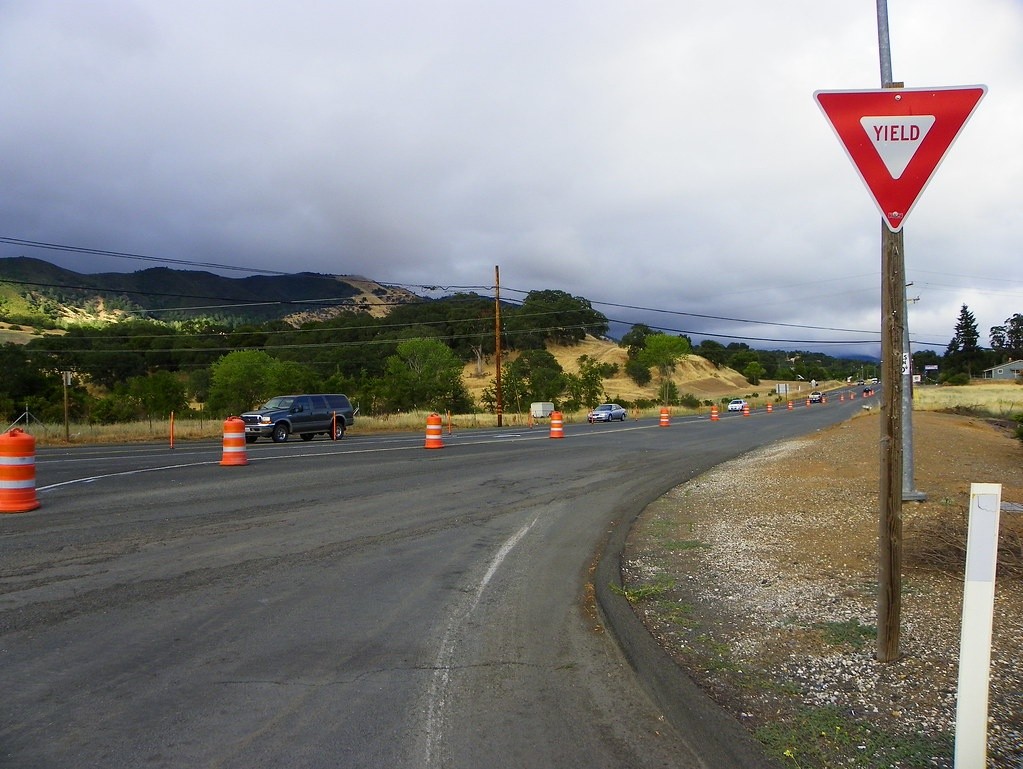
[864,386,874,393]
[587,404,627,423]
[858,380,865,385]
[728,399,748,411]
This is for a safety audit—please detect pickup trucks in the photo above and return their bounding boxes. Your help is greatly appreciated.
[808,391,825,403]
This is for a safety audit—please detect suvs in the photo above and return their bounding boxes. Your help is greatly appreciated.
[239,394,355,443]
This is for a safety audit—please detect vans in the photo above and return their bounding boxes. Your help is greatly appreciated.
[872,378,877,384]
